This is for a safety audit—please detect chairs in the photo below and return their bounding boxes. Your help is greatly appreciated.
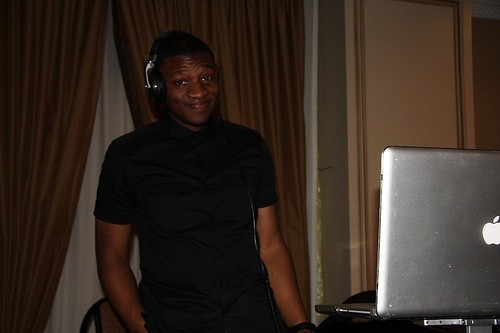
[79,289,147,333]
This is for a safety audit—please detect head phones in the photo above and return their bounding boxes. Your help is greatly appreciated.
[144,30,188,108]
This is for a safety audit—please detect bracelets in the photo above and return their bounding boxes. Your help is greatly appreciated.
[288,321,317,332]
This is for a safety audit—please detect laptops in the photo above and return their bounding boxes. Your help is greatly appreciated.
[314,145,500,326]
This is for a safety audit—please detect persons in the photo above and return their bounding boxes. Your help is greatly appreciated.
[94,29,318,333]
